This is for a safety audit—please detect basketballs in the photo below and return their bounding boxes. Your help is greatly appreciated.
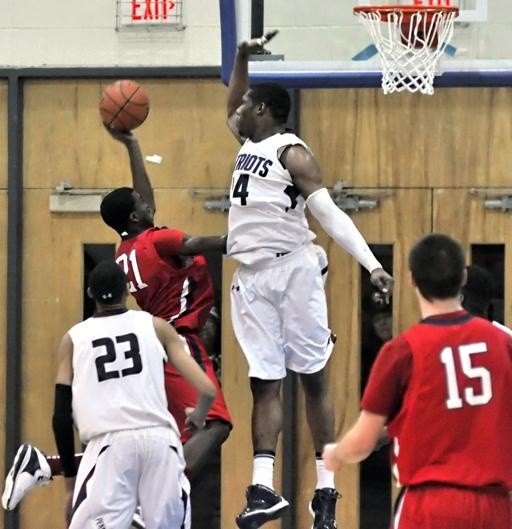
[99,79,150,133]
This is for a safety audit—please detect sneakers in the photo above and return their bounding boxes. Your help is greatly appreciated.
[1,444,52,511]
[308,488,339,529]
[236,484,290,529]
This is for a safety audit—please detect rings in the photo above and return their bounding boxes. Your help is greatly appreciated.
[256,36,268,45]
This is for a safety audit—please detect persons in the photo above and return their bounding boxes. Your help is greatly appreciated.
[225,30,394,529]
